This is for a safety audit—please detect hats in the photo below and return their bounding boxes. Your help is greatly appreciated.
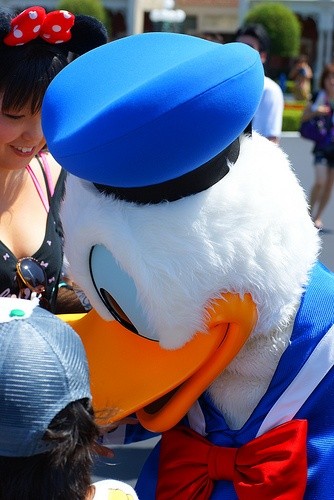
[0,297,92,457]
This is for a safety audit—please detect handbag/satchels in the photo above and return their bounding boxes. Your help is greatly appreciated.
[299,116,328,142]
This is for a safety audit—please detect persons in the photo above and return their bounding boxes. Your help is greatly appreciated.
[0,6,109,314]
[0,297,139,500]
[300,63,334,229]
[235,23,284,145]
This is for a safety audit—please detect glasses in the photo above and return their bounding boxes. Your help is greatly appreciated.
[16,256,52,313]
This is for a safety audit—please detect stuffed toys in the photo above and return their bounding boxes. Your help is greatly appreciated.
[40,32,334,500]
